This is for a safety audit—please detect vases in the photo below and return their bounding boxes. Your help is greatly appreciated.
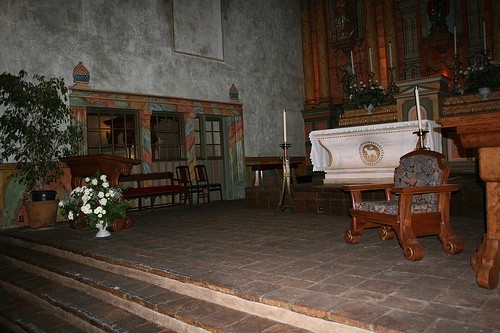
[458,85,464,95]
[365,104,374,114]
[477,86,492,101]
[95,220,111,238]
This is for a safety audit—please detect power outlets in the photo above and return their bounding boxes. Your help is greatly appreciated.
[18,215,24,222]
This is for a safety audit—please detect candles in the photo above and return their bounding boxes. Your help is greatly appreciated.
[453,24,457,55]
[388,41,393,67]
[369,46,373,72]
[414,85,422,129]
[283,108,287,142]
[350,50,354,74]
[483,19,487,50]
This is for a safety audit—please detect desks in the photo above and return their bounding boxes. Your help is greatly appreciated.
[457,119,500,290]
[58,154,136,232]
[246,160,303,187]
[309,120,443,184]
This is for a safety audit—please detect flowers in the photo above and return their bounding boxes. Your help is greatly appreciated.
[455,58,500,92]
[58,170,131,230]
[348,79,384,108]
[448,79,466,88]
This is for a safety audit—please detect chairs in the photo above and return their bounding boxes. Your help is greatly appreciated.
[175,165,205,207]
[194,164,223,205]
[341,149,464,262]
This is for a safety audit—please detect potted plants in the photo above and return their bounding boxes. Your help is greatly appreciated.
[0,69,88,230]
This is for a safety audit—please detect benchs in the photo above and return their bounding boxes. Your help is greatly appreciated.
[119,172,185,214]
